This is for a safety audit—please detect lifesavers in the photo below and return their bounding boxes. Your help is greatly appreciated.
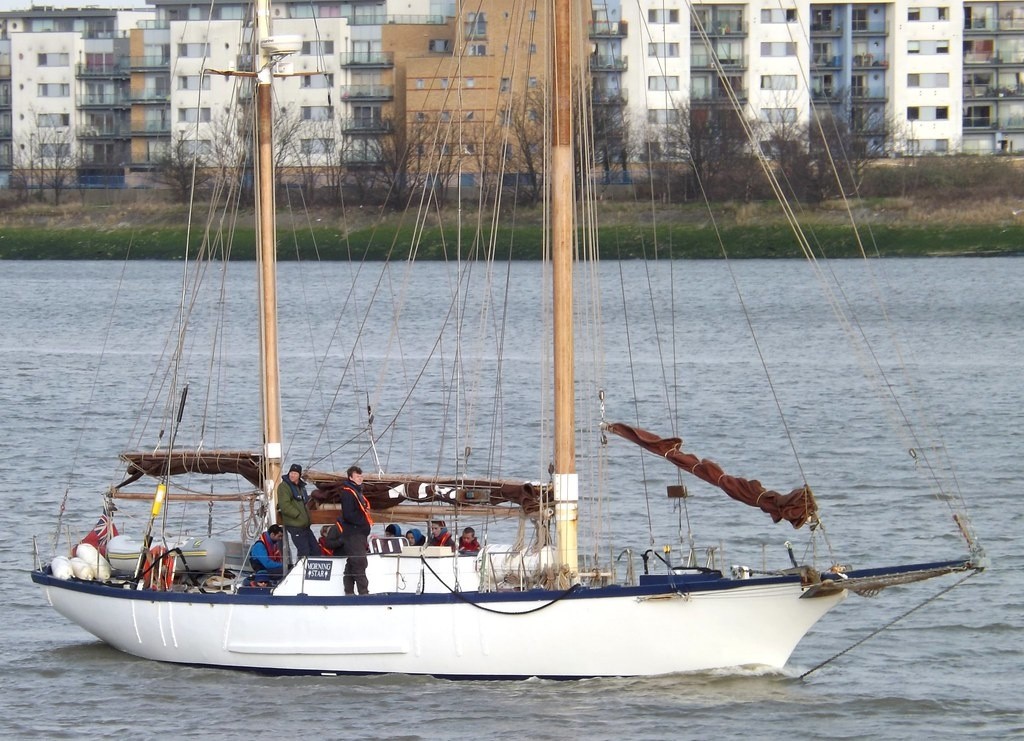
[143,546,173,591]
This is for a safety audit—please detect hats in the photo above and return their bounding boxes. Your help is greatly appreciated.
[385,524,396,535]
[287,463,302,477]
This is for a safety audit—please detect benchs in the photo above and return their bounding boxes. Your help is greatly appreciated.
[221,540,255,574]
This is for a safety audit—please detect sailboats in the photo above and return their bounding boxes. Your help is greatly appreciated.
[33,0,991,677]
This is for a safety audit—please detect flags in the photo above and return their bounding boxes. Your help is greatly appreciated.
[68,507,118,562]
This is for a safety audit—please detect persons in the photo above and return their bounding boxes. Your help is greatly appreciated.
[316,526,333,559]
[249,524,283,573]
[384,524,406,547]
[428,521,455,553]
[453,527,480,556]
[405,529,426,546]
[341,466,374,594]
[276,464,326,558]
